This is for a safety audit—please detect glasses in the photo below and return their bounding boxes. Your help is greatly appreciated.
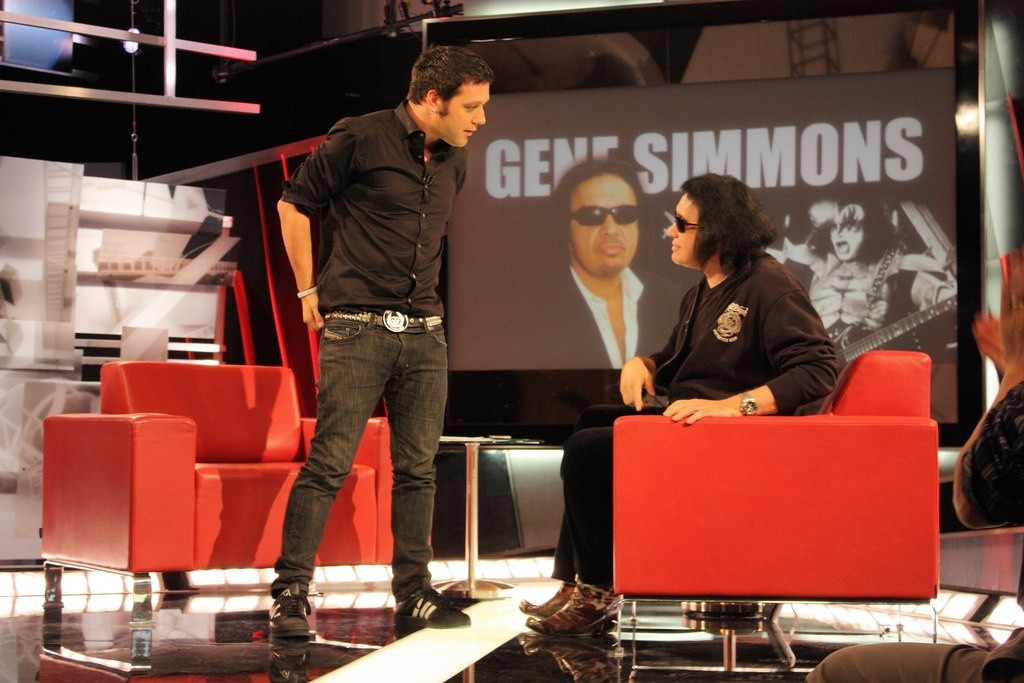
[674,210,700,233]
[567,204,641,226]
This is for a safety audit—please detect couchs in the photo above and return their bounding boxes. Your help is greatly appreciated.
[39,360,392,622]
[613,353,940,668]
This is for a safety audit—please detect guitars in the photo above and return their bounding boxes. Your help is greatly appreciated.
[832,295,957,373]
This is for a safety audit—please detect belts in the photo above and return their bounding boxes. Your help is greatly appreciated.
[324,308,443,333]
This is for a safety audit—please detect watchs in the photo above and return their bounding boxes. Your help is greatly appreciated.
[738,392,758,415]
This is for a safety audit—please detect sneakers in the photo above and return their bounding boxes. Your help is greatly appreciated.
[268,588,311,638]
[268,639,310,680]
[393,586,471,629]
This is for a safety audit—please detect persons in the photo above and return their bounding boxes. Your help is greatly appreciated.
[520,156,689,371]
[267,45,492,647]
[805,254,1024,683]
[519,634,622,683]
[516,174,836,636]
[783,192,958,347]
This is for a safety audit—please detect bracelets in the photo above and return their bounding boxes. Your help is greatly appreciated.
[298,286,317,298]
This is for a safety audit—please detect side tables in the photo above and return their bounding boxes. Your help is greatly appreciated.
[428,435,546,600]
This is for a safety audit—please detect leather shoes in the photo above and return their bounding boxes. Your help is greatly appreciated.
[526,588,623,638]
[519,582,577,616]
[517,632,621,683]
